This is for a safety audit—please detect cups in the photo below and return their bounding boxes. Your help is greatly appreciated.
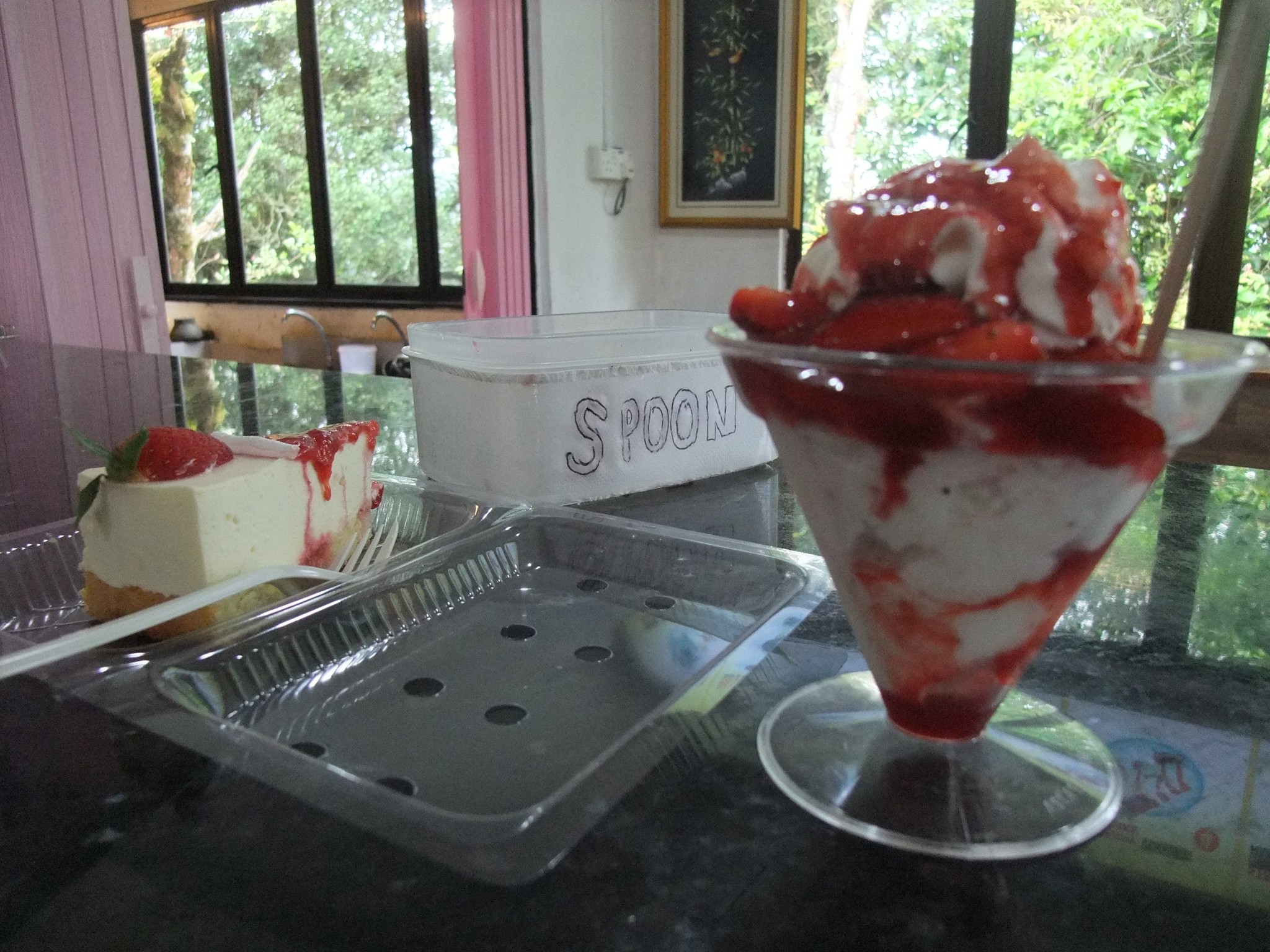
[704,307,1265,860]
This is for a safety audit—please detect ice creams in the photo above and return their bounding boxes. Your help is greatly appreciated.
[725,133,1179,742]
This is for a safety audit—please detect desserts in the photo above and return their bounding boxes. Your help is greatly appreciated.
[64,417,384,645]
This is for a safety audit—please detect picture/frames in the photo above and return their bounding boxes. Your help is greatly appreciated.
[657,0,806,230]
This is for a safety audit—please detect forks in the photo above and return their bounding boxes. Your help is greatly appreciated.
[3,513,408,693]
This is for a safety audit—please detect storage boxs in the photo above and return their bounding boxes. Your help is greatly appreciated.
[0,466,833,894]
[400,309,785,507]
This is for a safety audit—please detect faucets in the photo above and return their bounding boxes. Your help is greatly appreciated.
[372,312,412,377]
[281,309,335,371]
